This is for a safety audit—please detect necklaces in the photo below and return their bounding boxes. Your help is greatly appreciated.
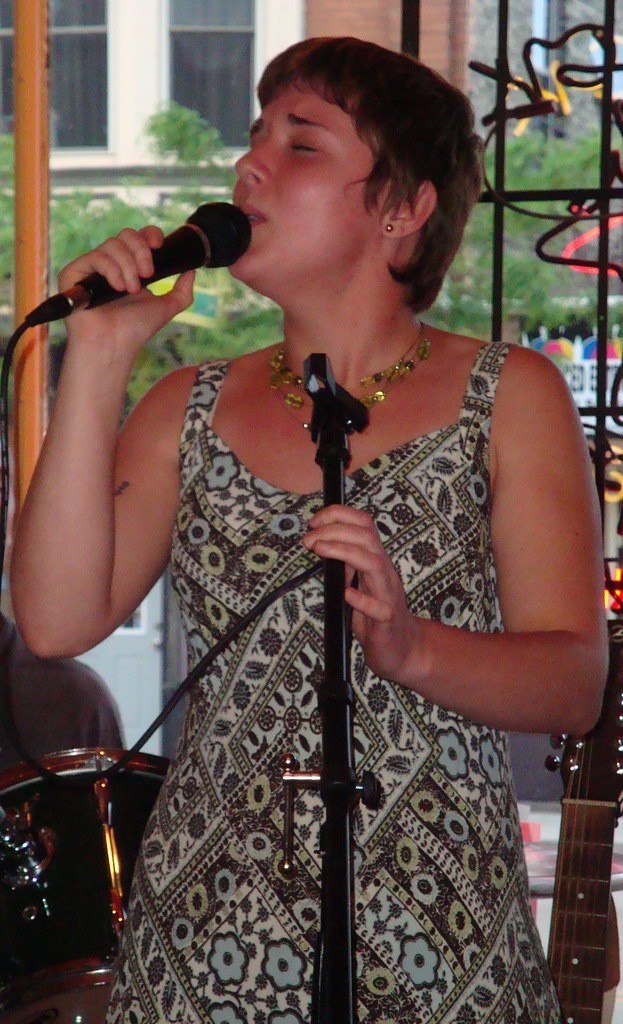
[262,323,430,443]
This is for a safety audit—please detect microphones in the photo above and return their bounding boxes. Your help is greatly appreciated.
[25,201,251,327]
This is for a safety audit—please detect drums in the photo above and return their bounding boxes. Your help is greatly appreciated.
[0,740,177,1024]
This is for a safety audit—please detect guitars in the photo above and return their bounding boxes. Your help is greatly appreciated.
[539,629,623,1024]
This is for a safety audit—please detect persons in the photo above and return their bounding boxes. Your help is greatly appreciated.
[0,613,125,771]
[7,33,607,1024]
